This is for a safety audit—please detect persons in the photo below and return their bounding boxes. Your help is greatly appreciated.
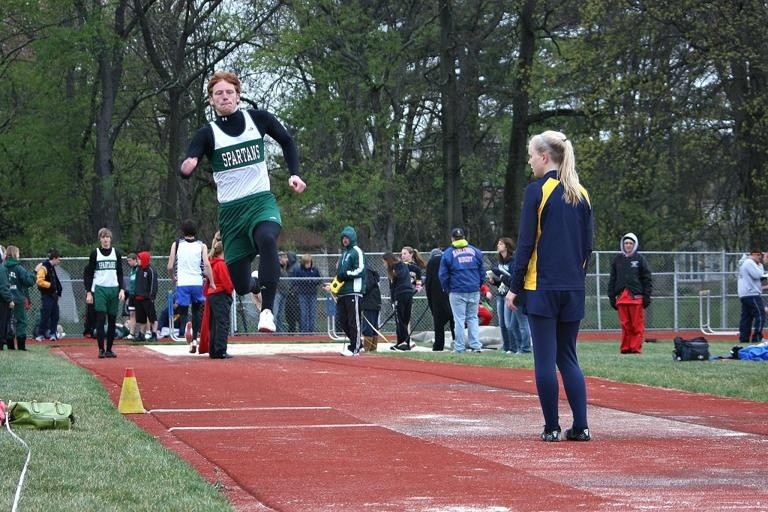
[507,130,593,442]
[737,249,768,343]
[178,70,307,333]
[607,232,652,354]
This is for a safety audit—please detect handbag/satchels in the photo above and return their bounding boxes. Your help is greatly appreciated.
[8,400,75,431]
[672,336,711,360]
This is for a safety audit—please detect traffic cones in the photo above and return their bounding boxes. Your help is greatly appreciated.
[115,367,146,413]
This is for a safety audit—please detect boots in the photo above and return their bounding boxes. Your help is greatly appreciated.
[363,336,378,351]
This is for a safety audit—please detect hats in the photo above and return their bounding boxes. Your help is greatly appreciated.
[451,228,466,239]
[127,252,137,258]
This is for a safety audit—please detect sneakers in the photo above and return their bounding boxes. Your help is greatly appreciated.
[189,340,197,353]
[50,333,57,340]
[540,430,562,442]
[98,348,105,358]
[563,428,591,441]
[36,335,45,341]
[106,350,117,357]
[122,331,157,342]
[339,347,365,356]
[390,340,416,350]
[258,309,276,332]
[250,270,263,311]
[210,353,234,358]
[185,322,194,343]
